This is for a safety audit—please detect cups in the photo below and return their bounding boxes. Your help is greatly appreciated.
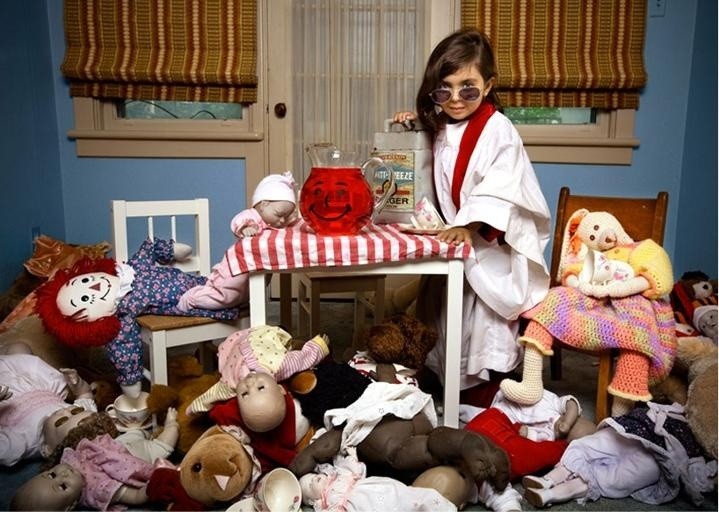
[300,142,395,235]
[253,466,303,511]
[105,391,152,427]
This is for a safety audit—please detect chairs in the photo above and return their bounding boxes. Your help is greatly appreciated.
[547,176,668,424]
[109,191,249,398]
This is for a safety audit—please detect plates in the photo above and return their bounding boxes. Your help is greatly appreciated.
[107,409,153,432]
[397,222,451,236]
[225,497,302,512]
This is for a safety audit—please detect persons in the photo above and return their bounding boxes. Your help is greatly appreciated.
[0,171,718,512]
[394,27,551,391]
[0,172,718,511]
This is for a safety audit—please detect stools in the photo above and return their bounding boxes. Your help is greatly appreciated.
[293,272,391,340]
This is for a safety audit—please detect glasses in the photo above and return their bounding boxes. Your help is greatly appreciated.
[428,79,489,104]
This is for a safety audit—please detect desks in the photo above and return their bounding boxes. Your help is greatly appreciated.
[230,219,477,430]
